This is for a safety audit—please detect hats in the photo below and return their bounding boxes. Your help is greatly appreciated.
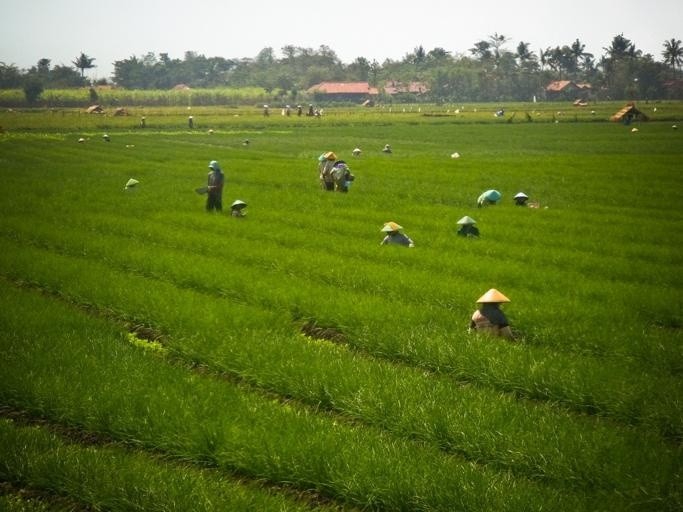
[231,200,247,209]
[514,192,528,200]
[208,160,218,171]
[476,289,511,303]
[126,178,139,186]
[457,215,476,225]
[381,222,403,232]
[353,148,361,153]
[486,190,501,200]
[319,152,345,167]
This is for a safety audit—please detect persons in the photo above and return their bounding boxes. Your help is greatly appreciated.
[229,200,247,217]
[512,192,528,206]
[329,160,353,193]
[307,104,313,115]
[188,116,193,129]
[317,152,326,189]
[296,105,302,117]
[102,135,109,142]
[285,104,290,117]
[262,105,270,117]
[322,152,337,191]
[140,116,145,128]
[378,221,414,249]
[381,144,392,153]
[204,161,224,214]
[77,138,85,144]
[467,289,513,340]
[351,148,362,158]
[455,216,480,238]
[124,178,139,191]
[476,190,502,209]
[493,108,504,117]
